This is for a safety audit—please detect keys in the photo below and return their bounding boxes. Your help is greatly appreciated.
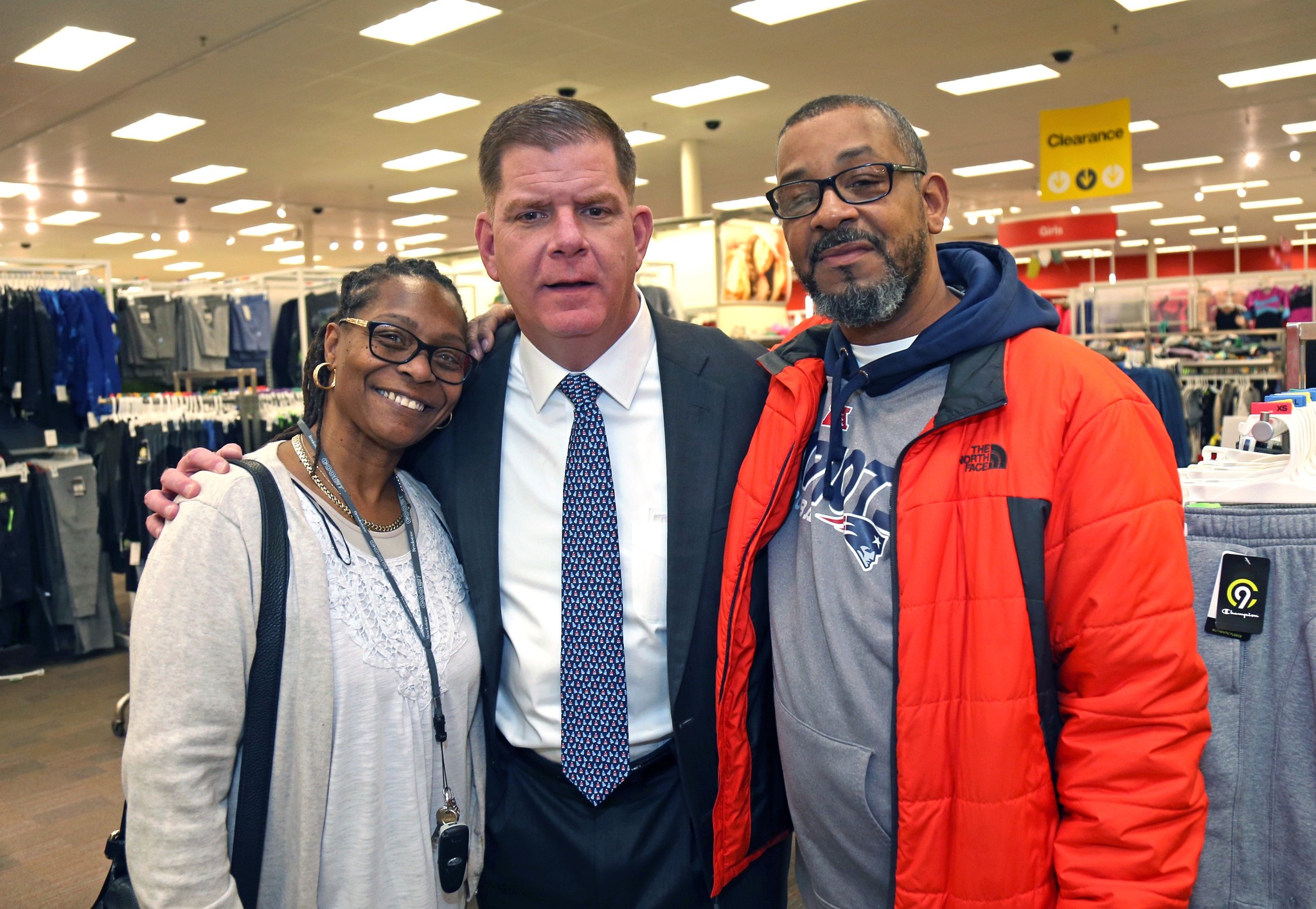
[432,802,469,893]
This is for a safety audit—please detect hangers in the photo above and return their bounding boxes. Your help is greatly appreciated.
[1179,374,1261,397]
[1154,275,1311,315]
[1176,401,1316,537]
[1087,341,1172,382]
[0,267,304,483]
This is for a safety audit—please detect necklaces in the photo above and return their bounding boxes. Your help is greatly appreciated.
[290,432,408,533]
[300,433,343,498]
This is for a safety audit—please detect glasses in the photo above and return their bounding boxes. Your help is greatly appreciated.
[339,316,481,386]
[766,164,925,219]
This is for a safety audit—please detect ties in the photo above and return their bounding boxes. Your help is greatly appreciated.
[559,372,631,805]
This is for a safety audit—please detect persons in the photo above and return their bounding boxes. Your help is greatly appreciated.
[120,258,493,909]
[144,95,773,909]
[464,95,1211,909]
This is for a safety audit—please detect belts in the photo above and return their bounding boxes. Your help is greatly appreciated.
[495,729,681,796]
